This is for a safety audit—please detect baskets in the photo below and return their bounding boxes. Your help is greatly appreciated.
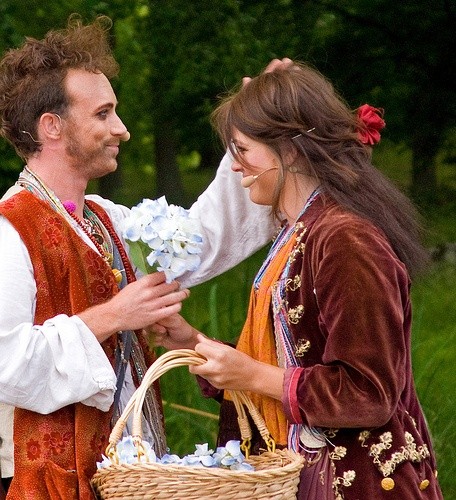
[91,349,306,499]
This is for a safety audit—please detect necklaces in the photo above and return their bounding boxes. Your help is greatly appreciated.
[58,199,133,369]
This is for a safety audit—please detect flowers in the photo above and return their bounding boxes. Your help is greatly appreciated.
[96,436,254,472]
[119,195,204,355]
[355,104,387,145]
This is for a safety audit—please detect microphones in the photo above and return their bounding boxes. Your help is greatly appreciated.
[241,168,297,187]
[121,132,130,141]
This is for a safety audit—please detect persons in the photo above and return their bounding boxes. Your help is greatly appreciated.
[142,62,444,500]
[0,12,302,500]
